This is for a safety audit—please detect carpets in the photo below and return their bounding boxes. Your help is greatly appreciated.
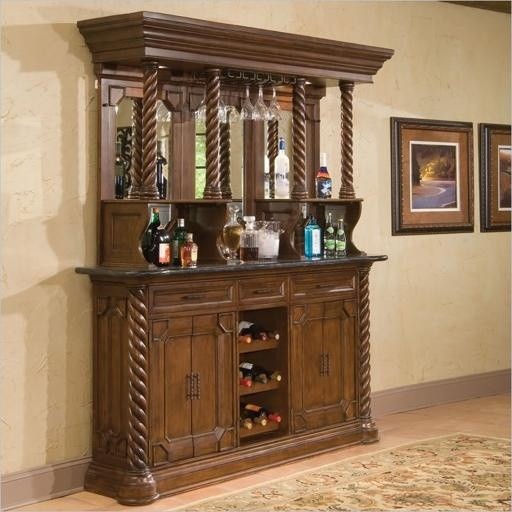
[161,433,512,512]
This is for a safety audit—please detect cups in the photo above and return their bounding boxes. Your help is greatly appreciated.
[265,80,283,123]
[256,221,281,263]
[230,104,240,123]
[240,79,258,119]
[251,80,269,121]
[156,103,171,123]
[218,76,228,122]
[197,80,206,120]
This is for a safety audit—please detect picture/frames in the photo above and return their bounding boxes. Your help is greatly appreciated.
[392,116,474,236]
[478,122,512,235]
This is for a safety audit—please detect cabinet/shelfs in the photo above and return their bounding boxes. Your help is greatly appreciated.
[71,10,388,506]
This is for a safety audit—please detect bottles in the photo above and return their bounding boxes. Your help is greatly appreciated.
[243,403,281,423]
[336,219,346,258]
[156,141,168,199]
[242,363,281,382]
[153,226,170,266]
[324,213,337,259]
[316,152,332,198]
[242,321,280,341]
[274,138,289,199]
[304,217,321,260]
[241,417,252,429]
[239,373,267,386]
[181,234,197,268]
[113,144,126,199]
[147,208,163,265]
[171,219,188,266]
[240,216,260,264]
[241,409,266,426]
[240,328,267,344]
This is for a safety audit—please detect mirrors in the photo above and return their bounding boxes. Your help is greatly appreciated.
[193,98,245,200]
[264,109,293,200]
[113,94,174,200]
